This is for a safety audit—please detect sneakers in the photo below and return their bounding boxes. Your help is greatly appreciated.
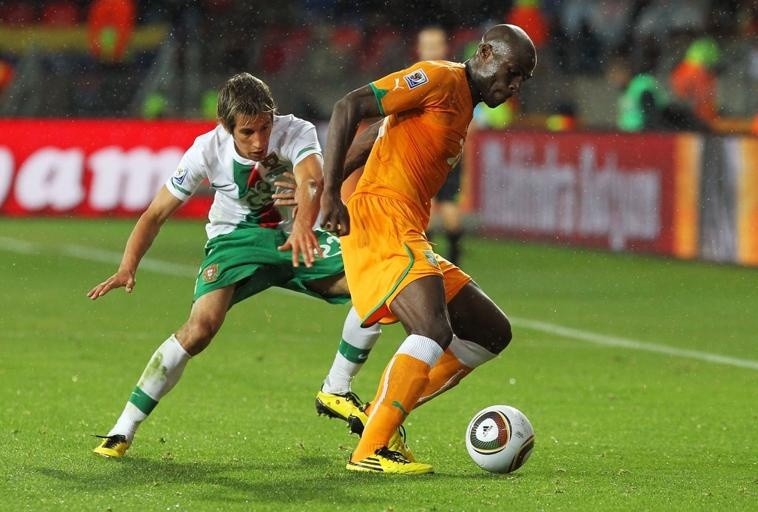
[91,431,132,462]
[345,401,420,464]
[314,383,362,424]
[346,444,435,477]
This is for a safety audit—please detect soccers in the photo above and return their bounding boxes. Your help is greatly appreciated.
[465,405,535,474]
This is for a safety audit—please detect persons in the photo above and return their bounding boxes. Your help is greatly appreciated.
[1,0,756,132]
[271,20,537,475]
[84,72,381,461]
